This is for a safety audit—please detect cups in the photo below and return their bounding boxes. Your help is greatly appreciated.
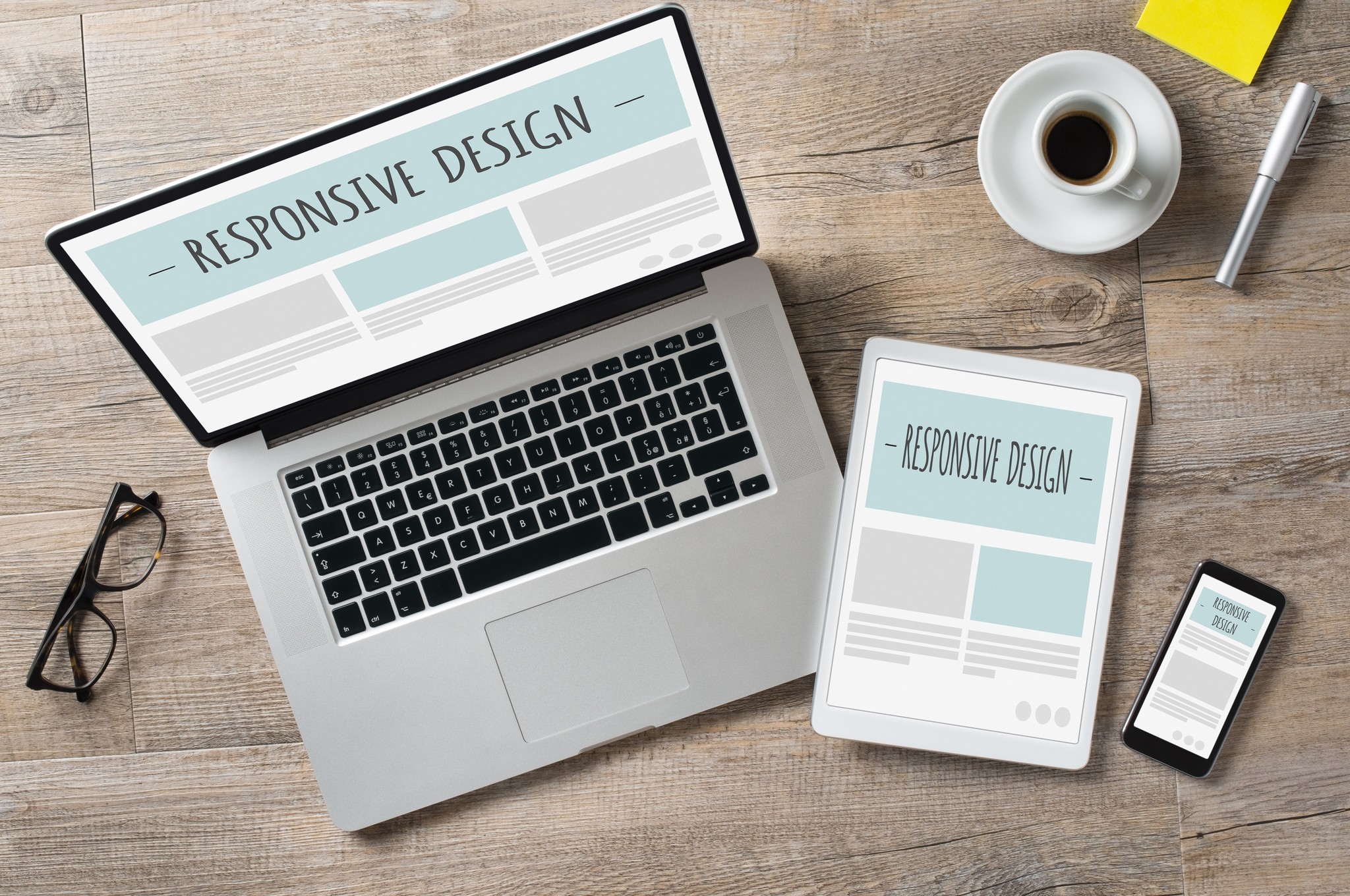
[1031,89,1151,202]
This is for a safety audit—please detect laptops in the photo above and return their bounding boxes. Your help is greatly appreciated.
[42,5,845,832]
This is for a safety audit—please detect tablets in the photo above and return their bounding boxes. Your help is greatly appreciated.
[809,332,1144,772]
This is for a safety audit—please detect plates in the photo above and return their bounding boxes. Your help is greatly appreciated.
[977,49,1181,254]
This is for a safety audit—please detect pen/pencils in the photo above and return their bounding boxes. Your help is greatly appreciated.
[1214,83,1323,291]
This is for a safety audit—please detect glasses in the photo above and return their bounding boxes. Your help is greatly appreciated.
[23,482,167,704]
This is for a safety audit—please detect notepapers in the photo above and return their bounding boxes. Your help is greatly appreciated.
[1135,0,1293,86]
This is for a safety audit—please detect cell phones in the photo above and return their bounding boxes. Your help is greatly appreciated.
[1120,556,1287,780]
